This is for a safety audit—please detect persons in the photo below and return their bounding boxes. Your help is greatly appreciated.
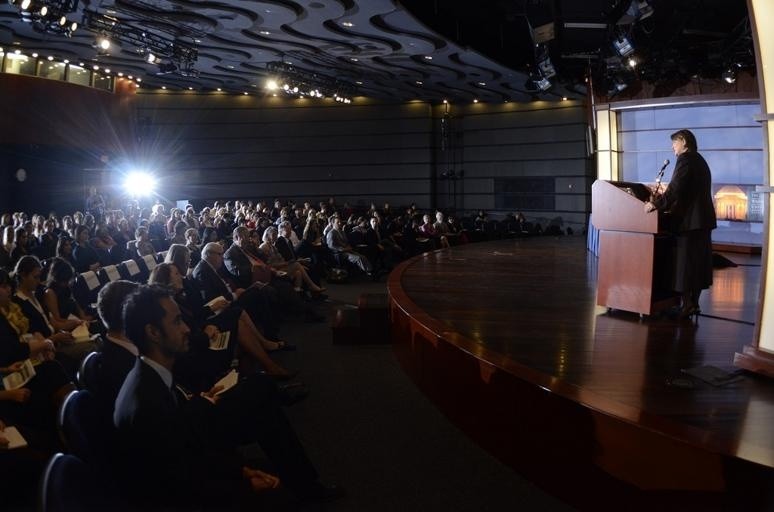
[86,185,106,210]
[644,129,717,321]
[0,198,529,512]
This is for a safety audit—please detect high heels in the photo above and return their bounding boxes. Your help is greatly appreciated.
[678,305,702,323]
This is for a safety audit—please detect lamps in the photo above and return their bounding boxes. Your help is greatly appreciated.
[0,0,353,105]
[721,64,737,84]
[522,0,655,99]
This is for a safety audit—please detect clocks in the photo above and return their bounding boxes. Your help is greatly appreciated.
[15,168,28,184]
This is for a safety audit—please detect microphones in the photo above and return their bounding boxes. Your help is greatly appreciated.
[657,160,670,176]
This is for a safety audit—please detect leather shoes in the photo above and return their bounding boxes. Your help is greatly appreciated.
[313,486,346,500]
[264,285,327,378]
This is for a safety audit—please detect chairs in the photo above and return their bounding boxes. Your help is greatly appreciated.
[55,388,109,451]
[156,251,168,264]
[41,452,103,512]
[74,270,102,316]
[138,254,159,276]
[76,350,118,396]
[96,264,123,286]
[121,259,146,285]
